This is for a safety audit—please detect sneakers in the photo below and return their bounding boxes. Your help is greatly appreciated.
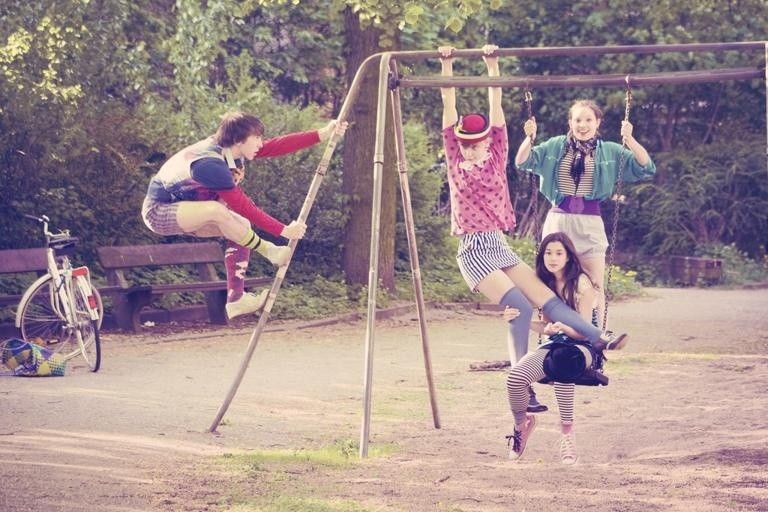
[225,288,270,321]
[270,245,292,266]
[559,429,579,466]
[505,413,538,462]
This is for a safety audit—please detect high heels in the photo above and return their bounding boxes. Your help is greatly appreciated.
[525,385,548,412]
[592,329,629,362]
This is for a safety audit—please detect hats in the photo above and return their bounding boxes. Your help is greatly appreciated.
[453,112,492,145]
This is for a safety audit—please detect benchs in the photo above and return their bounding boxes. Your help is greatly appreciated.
[0,240,274,335]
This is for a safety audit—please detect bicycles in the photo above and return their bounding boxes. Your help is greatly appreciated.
[14,211,103,373]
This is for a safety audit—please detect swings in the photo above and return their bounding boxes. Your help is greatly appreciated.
[524,74,632,386]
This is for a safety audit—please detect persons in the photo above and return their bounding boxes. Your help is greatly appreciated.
[436,45,628,411]
[515,98,658,374]
[504,233,598,466]
[141,112,348,320]
